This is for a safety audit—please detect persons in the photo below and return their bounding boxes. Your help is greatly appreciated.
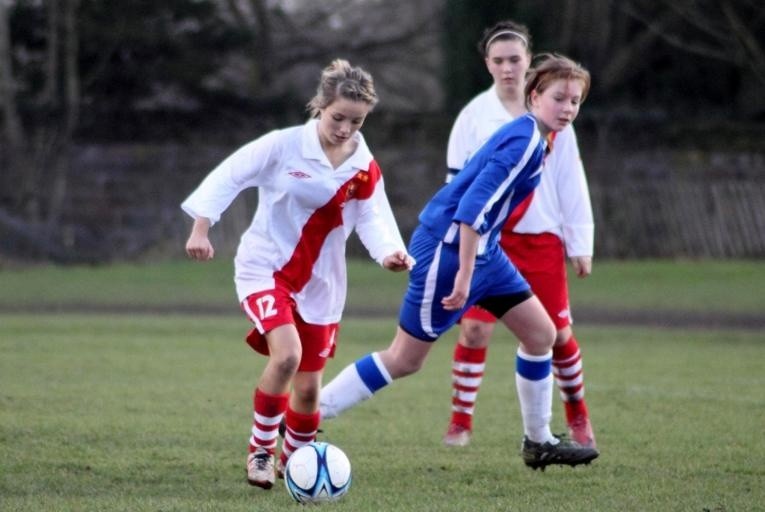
[281,53,600,468]
[445,18,596,453]
[179,58,416,488]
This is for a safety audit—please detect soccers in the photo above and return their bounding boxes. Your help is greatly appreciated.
[284,442,351,504]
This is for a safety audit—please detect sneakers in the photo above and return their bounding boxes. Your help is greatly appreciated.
[567,415,596,448]
[522,436,598,467]
[445,423,468,446]
[247,450,276,490]
